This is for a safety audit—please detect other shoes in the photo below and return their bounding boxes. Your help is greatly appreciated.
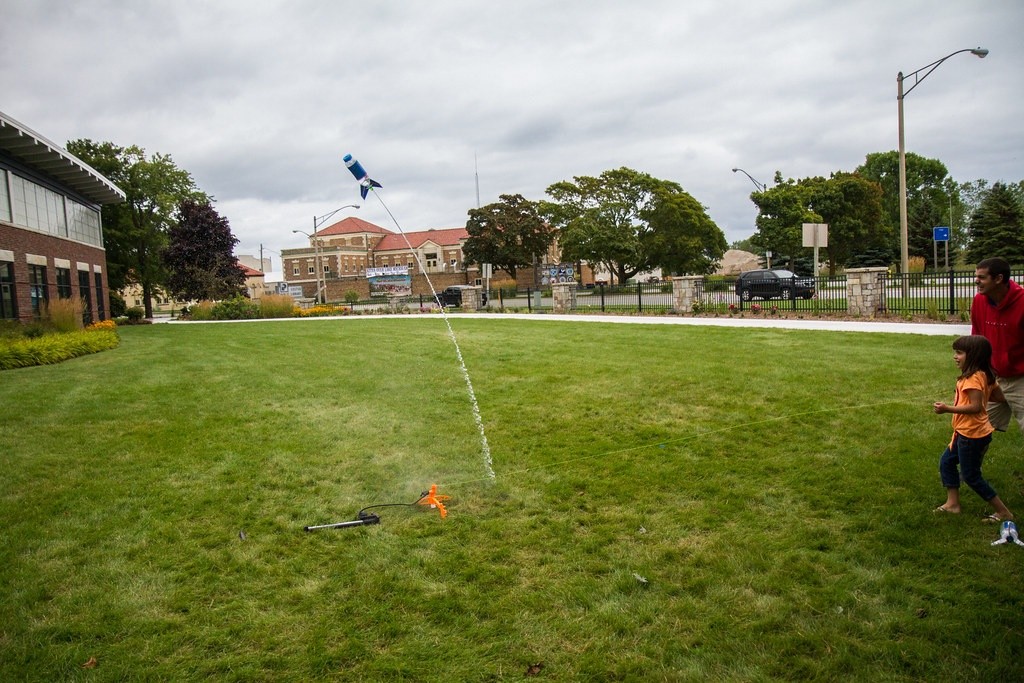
[981,512,1014,522]
[932,505,961,514]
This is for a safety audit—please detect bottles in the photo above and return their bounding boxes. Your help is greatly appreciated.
[343,153,374,191]
[999,520,1018,544]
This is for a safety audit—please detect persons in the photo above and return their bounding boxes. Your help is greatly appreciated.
[933,336,1013,524]
[947,258,1024,480]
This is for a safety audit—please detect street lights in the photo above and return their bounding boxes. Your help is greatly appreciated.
[313,203,360,306]
[292,230,329,304]
[896,44,991,297]
[731,166,767,193]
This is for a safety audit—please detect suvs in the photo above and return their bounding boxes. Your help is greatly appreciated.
[733,269,817,302]
[431,285,487,307]
